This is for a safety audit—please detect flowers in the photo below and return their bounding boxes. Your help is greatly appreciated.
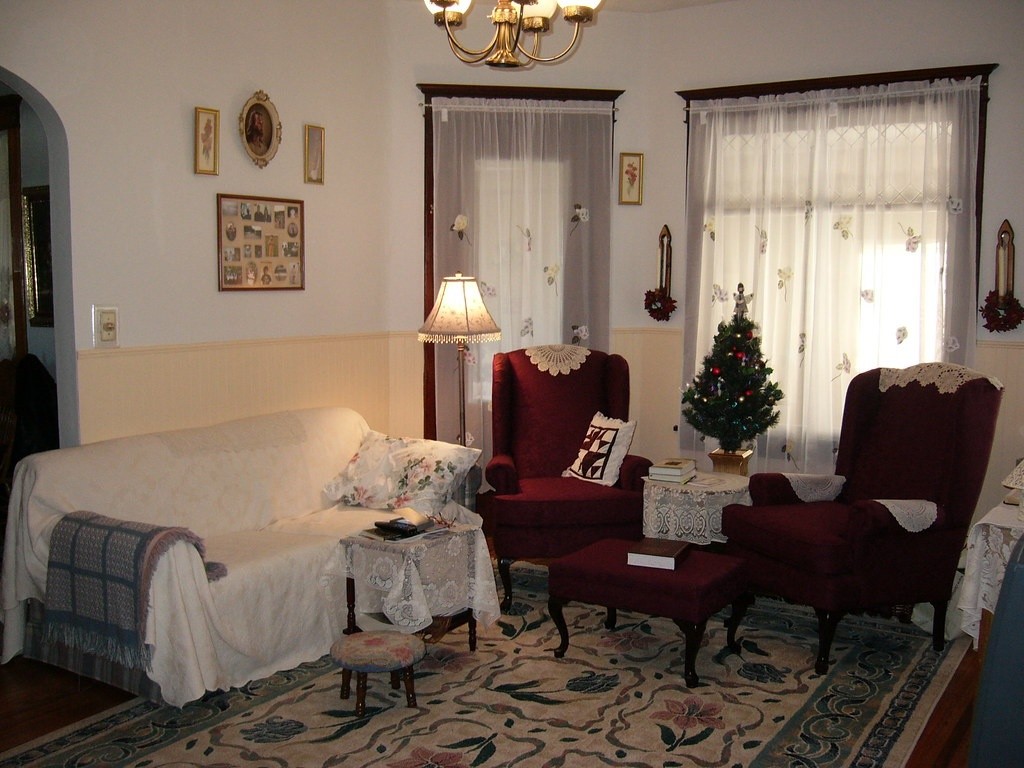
[979,290,1024,334]
[644,286,678,322]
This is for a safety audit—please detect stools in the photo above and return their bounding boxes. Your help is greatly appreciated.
[330,628,430,720]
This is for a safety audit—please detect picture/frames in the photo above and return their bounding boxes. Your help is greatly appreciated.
[21,184,55,328]
[237,89,283,169]
[216,193,306,291]
[619,152,644,205]
[305,124,325,185]
[195,107,221,177]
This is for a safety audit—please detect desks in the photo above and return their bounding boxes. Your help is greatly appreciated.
[451,463,482,514]
[641,471,754,552]
[544,537,758,691]
[975,505,1024,688]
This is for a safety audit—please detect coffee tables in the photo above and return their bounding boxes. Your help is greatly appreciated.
[319,518,502,655]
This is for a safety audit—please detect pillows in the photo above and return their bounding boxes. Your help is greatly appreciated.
[322,430,483,518]
[559,410,637,488]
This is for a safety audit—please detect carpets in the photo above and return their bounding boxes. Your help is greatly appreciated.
[0,558,975,768]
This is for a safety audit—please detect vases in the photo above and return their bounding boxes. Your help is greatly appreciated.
[709,447,754,477]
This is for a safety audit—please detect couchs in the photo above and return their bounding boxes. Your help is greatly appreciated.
[0,404,483,719]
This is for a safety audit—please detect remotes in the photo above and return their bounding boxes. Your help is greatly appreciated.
[374,521,417,537]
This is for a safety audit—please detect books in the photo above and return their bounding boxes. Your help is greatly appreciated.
[648,458,697,483]
[626,537,690,570]
[360,527,400,542]
[1003,488,1021,505]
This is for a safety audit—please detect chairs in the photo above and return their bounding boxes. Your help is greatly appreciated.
[721,361,1002,677]
[485,343,654,612]
[957,532,1024,768]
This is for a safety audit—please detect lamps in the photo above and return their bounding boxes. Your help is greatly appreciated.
[421,0,603,68]
[417,269,504,448]
